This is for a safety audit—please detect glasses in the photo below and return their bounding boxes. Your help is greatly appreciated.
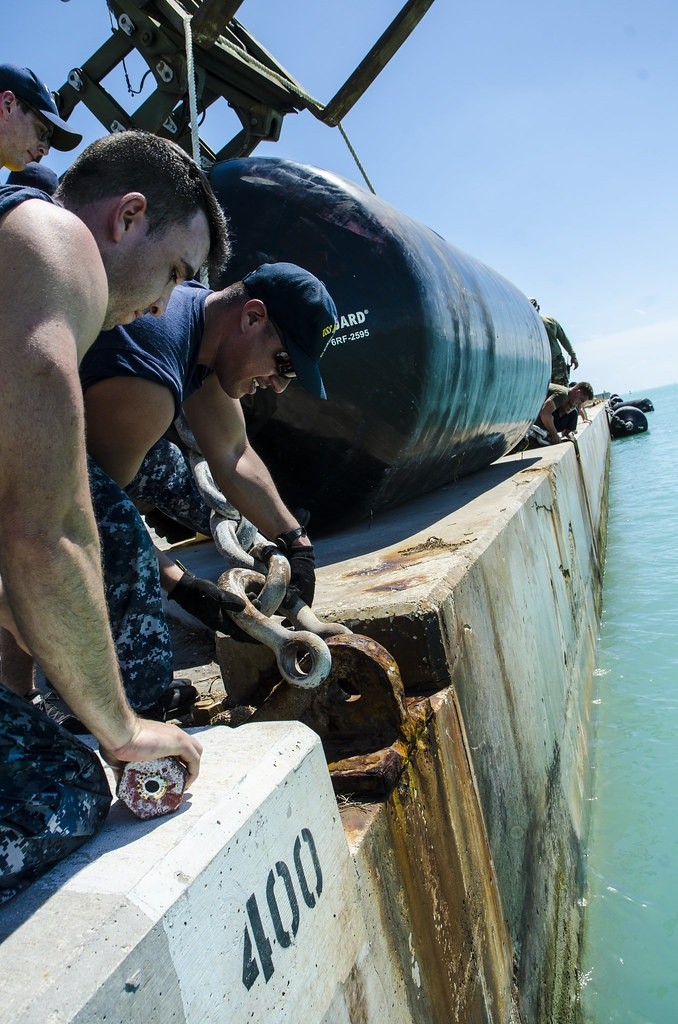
[273,351,299,380]
[15,95,53,141]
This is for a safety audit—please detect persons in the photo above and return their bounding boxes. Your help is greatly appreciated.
[0,61,84,174]
[568,382,592,424]
[78,260,344,644]
[0,130,234,906]
[4,160,61,198]
[528,297,579,387]
[535,382,594,444]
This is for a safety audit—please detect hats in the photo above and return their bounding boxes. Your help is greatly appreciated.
[0,64,83,152]
[243,262,341,401]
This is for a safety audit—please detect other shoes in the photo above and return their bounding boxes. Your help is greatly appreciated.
[62,678,201,733]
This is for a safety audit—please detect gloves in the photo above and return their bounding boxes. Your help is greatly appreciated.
[277,544,315,628]
[167,570,262,644]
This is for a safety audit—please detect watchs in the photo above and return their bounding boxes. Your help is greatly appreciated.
[274,526,307,551]
